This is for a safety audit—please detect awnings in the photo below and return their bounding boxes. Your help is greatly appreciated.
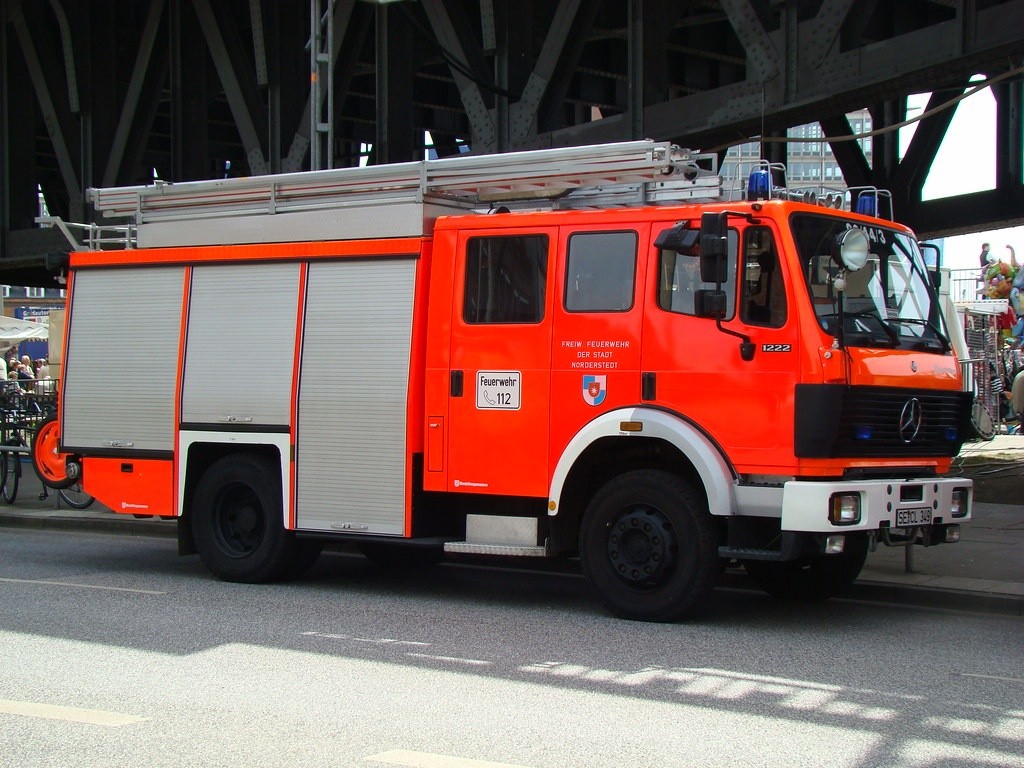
[0,315,49,346]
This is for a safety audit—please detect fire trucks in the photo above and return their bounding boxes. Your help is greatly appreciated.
[31,134,974,624]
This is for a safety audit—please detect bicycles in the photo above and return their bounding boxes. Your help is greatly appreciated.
[1,390,95,510]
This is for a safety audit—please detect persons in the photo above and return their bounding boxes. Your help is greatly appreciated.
[0,351,50,413]
[979,243,991,300]
